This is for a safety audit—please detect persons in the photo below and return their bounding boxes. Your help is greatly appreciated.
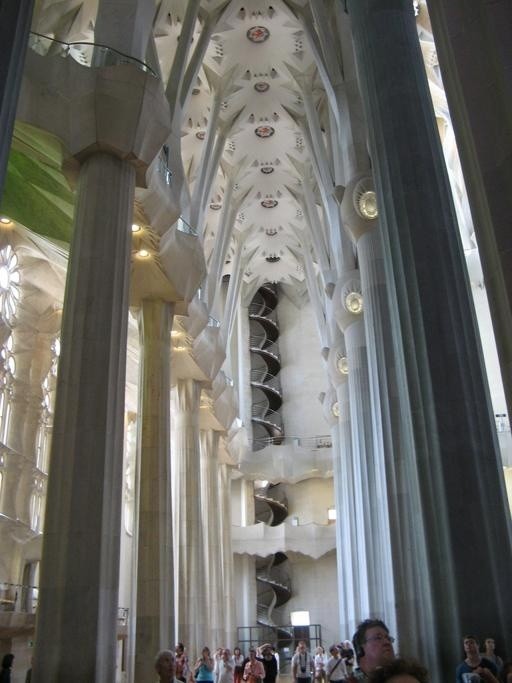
[0,654,15,683]
[26,658,32,683]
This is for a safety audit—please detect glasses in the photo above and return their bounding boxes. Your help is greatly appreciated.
[364,635,394,643]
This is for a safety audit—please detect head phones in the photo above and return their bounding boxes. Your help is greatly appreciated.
[353,628,365,657]
